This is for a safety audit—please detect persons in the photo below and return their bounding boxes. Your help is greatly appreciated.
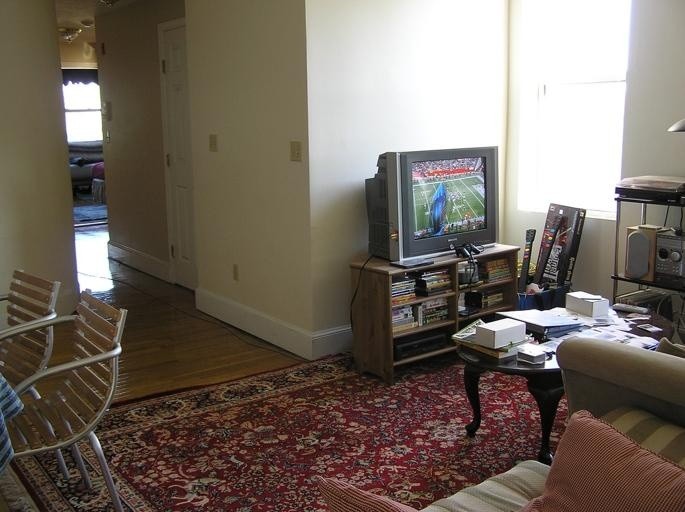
[410,157,485,240]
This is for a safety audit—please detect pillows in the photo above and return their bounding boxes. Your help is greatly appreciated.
[520,409,685,512]
[314,475,419,512]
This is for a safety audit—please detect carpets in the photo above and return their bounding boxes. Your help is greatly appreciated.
[0,351,570,512]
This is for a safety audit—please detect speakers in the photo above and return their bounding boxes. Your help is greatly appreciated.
[624,224,667,283]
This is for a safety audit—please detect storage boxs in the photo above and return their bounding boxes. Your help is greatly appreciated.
[517,282,572,311]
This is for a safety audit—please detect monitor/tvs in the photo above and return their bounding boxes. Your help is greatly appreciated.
[364,146,500,262]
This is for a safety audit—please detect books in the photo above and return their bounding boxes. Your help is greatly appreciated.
[389,256,514,336]
[451,317,534,352]
[525,325,585,342]
[453,339,540,360]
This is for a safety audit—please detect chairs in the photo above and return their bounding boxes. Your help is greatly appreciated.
[0,270,71,481]
[416,336,685,512]
[0,288,128,512]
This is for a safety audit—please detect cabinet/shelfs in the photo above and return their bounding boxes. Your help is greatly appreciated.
[610,196,685,305]
[348,243,522,386]
